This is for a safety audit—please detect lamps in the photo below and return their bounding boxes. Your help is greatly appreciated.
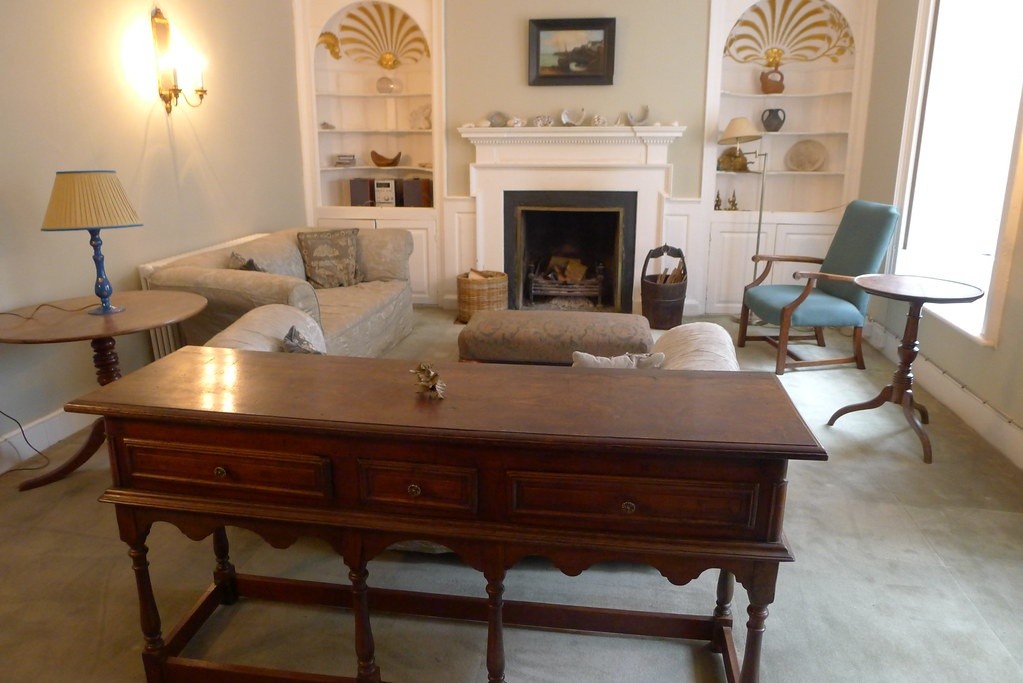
[41,170,144,315]
[718,117,768,325]
[151,9,207,115]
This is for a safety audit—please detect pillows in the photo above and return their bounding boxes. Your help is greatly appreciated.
[572,351,665,368]
[229,251,268,272]
[297,228,368,289]
[281,326,326,355]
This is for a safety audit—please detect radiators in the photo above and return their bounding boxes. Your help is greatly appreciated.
[139,233,269,361]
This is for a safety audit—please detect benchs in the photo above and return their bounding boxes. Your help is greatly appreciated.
[458,310,654,365]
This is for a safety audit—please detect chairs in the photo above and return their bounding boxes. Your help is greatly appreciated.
[736,200,901,375]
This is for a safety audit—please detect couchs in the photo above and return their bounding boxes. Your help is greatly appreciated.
[150,228,414,357]
[204,305,739,371]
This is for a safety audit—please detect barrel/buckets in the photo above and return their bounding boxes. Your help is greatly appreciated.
[641,244,687,331]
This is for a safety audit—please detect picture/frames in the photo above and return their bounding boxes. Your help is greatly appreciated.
[528,17,616,86]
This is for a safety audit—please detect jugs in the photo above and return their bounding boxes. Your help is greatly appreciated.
[761,109,785,131]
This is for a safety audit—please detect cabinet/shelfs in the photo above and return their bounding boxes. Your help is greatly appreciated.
[292,0,447,306]
[698,0,878,318]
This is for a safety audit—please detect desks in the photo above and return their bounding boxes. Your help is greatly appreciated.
[0,290,208,491]
[828,273,984,463]
[65,345,829,683]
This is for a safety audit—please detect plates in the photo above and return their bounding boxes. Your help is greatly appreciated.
[786,140,826,171]
